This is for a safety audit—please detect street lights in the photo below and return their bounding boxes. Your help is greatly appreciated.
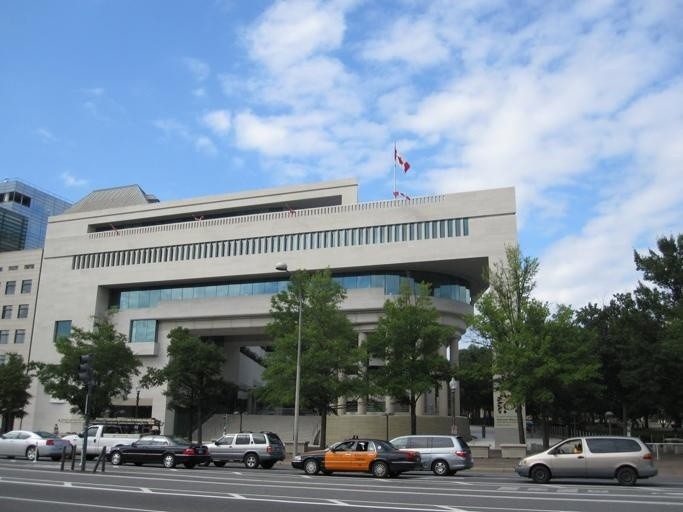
[381,412,392,442]
[449,377,457,434]
[276,263,303,455]
[233,407,248,432]
[135,383,142,417]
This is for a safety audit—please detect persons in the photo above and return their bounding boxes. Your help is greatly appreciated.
[574,442,582,453]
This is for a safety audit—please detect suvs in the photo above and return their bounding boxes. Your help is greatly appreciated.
[378,435,473,476]
[197,431,286,469]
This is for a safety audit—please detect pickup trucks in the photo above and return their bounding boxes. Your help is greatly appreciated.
[60,424,155,461]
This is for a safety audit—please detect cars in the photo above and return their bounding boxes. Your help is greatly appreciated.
[0,430,72,461]
[108,434,211,469]
[291,435,422,478]
[515,436,658,486]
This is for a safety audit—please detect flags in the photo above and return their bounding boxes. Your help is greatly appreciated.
[392,191,411,201]
[394,150,410,174]
